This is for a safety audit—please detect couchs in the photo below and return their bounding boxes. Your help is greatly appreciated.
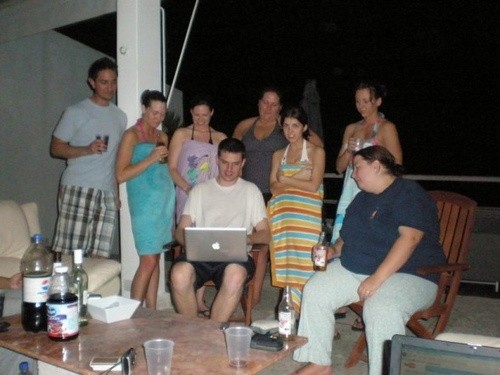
[0,199,121,317]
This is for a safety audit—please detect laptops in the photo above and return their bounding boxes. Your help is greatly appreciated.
[184,226,250,263]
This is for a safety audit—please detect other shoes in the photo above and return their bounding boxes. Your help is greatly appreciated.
[333,331,341,340]
[351,318,365,331]
[334,312,347,319]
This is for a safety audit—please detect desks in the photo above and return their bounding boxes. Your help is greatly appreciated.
[1,307,308,375]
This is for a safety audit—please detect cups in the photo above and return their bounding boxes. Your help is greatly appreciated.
[157,142,165,162]
[224,327,253,368]
[143,339,175,375]
[354,138,362,151]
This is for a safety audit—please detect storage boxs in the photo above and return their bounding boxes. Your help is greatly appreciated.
[86,295,140,322]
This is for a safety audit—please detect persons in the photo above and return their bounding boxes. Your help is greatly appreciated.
[293,146,445,375]
[115,90,176,309]
[337,84,403,332]
[0,273,22,289]
[50,57,128,258]
[170,138,270,322]
[266,107,341,341]
[169,96,227,225]
[232,88,324,306]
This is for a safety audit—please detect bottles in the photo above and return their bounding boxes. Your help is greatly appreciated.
[67,249,88,327]
[48,262,62,292]
[277,285,296,342]
[313,231,327,272]
[47,266,80,341]
[21,234,53,333]
[18,361,34,375]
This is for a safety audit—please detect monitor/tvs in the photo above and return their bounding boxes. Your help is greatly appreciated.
[389,333,500,375]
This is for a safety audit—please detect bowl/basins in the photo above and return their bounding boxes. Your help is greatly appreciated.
[85,295,141,323]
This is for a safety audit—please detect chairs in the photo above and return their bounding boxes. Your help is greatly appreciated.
[162,241,262,326]
[343,191,477,368]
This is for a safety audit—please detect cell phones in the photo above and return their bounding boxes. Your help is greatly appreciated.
[249,334,283,351]
[122,346,137,375]
